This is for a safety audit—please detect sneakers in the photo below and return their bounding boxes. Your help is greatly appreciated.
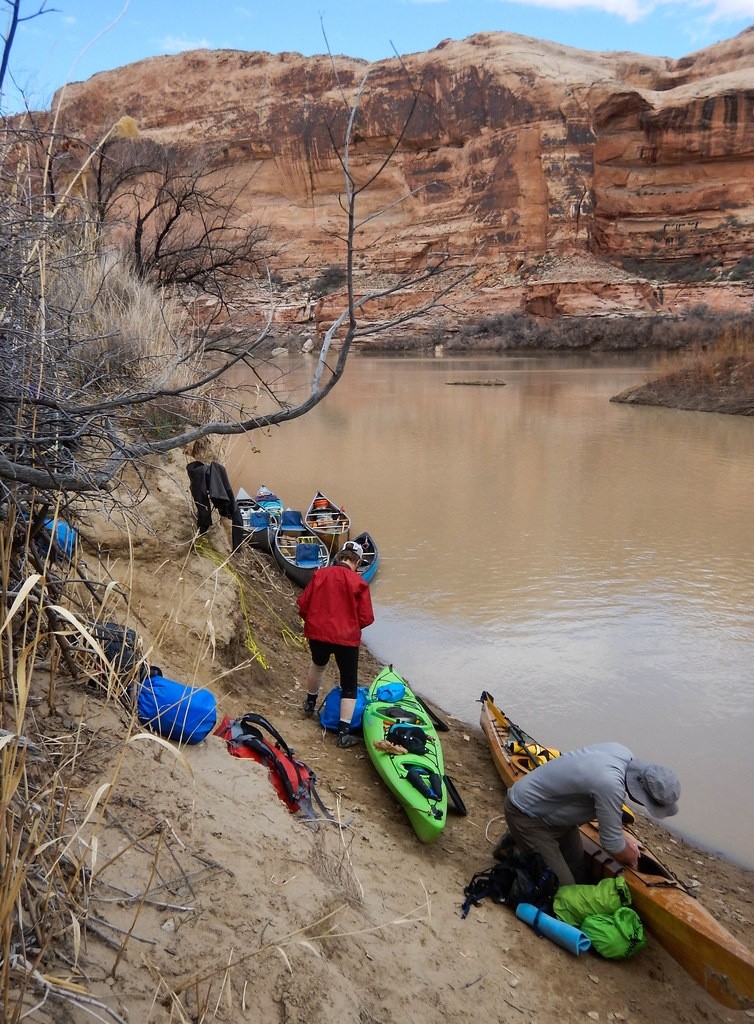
[302,700,315,716]
[336,730,358,747]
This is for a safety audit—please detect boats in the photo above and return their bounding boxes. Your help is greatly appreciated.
[229,479,379,597]
[480,691,754,1011]
[363,663,448,846]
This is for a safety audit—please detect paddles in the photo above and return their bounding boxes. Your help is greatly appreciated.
[485,695,542,767]
[329,505,344,554]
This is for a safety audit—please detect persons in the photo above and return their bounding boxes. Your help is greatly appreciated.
[504,742,679,890]
[298,542,374,748]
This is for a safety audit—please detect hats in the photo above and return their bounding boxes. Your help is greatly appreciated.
[625,756,682,819]
[342,540,364,558]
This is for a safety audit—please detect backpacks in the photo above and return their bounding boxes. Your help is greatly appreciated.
[487,852,646,960]
[317,684,366,735]
[101,623,314,813]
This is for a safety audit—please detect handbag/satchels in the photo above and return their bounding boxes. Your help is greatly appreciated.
[387,727,426,756]
[377,683,405,703]
